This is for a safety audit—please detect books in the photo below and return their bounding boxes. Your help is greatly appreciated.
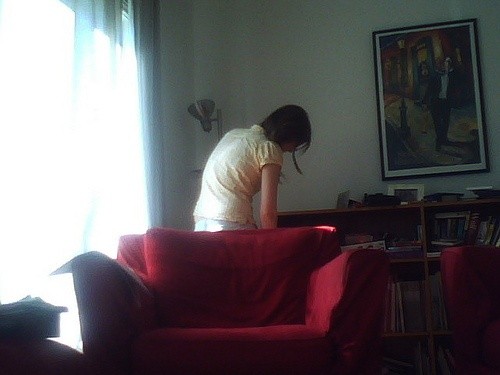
[343,208,500,375]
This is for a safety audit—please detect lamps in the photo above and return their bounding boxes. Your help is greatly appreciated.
[188,98,222,141]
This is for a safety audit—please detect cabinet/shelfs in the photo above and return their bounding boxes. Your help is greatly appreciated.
[278,198,500,375]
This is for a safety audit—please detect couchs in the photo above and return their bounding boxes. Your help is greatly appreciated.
[440,244,500,375]
[70,226,388,375]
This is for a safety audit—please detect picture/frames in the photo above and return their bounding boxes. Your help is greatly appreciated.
[371,17,490,181]
[388,184,424,202]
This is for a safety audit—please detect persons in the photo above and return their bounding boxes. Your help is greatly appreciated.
[192,105,311,231]
